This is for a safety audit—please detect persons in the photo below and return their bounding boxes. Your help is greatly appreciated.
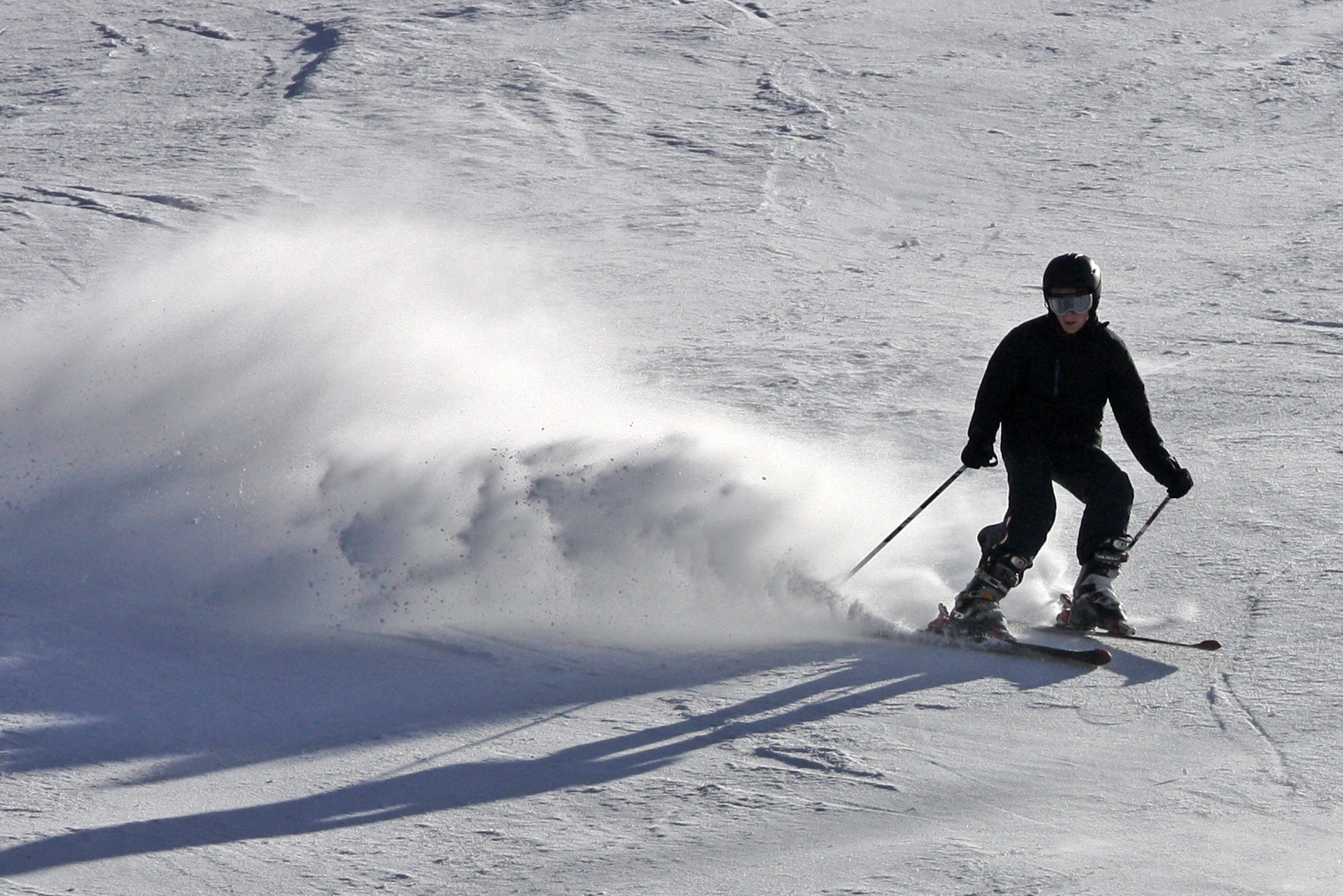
[951,252,1192,633]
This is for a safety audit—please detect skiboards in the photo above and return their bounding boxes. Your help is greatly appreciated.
[847,592,1223,666]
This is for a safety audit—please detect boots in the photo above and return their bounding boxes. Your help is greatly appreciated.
[1073,559,1129,623]
[949,545,1032,643]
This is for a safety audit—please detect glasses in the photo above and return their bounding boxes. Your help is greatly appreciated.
[1049,293,1095,315]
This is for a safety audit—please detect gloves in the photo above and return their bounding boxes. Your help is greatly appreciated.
[960,437,995,468]
[1155,463,1195,500]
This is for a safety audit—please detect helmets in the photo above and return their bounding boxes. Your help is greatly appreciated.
[1042,254,1102,317]
